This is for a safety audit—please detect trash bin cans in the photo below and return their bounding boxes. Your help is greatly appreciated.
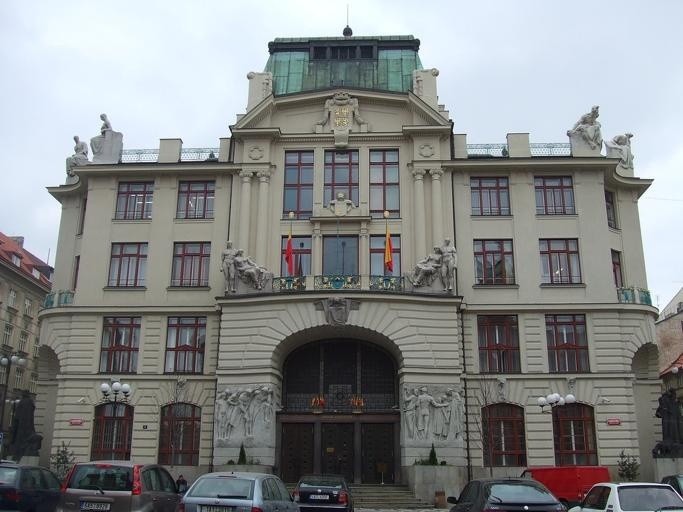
[435,491,445,509]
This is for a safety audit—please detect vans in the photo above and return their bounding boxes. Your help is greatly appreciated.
[520,466,612,509]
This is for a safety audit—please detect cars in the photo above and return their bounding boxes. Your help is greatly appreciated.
[446,475,683,512]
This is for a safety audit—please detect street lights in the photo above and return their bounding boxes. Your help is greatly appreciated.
[100,382,131,460]
[538,393,576,466]
[0,355,27,430]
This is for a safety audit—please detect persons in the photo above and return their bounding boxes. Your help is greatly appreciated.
[176,475,187,486]
[611,132,634,169]
[90,114,113,155]
[567,104,601,149]
[219,241,266,292]
[215,386,273,439]
[404,386,463,440]
[655,386,683,445]
[9,389,36,461]
[411,238,457,292]
[64,136,88,176]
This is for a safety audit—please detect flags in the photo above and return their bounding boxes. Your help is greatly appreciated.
[284,222,293,274]
[385,227,393,272]
